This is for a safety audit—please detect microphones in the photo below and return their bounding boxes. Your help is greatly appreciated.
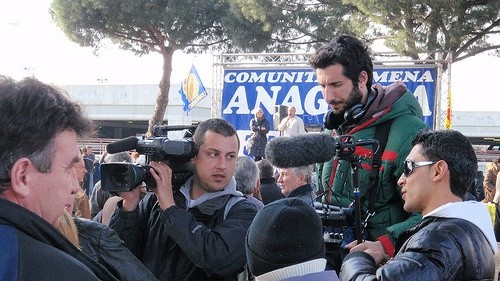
[264,134,377,169]
[106,137,140,154]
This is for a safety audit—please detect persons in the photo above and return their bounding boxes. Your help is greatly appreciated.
[0,35,500,281]
[245,198,339,281]
[0,75,119,281]
[340,129,498,281]
[278,106,305,136]
[109,119,257,281]
[248,108,270,162]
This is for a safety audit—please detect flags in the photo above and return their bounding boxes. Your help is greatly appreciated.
[179,65,207,115]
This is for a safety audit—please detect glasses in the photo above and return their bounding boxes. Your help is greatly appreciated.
[403,159,437,176]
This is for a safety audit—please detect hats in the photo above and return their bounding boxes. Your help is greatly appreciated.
[245,197,325,276]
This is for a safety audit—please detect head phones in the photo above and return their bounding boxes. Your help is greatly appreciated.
[322,88,378,130]
